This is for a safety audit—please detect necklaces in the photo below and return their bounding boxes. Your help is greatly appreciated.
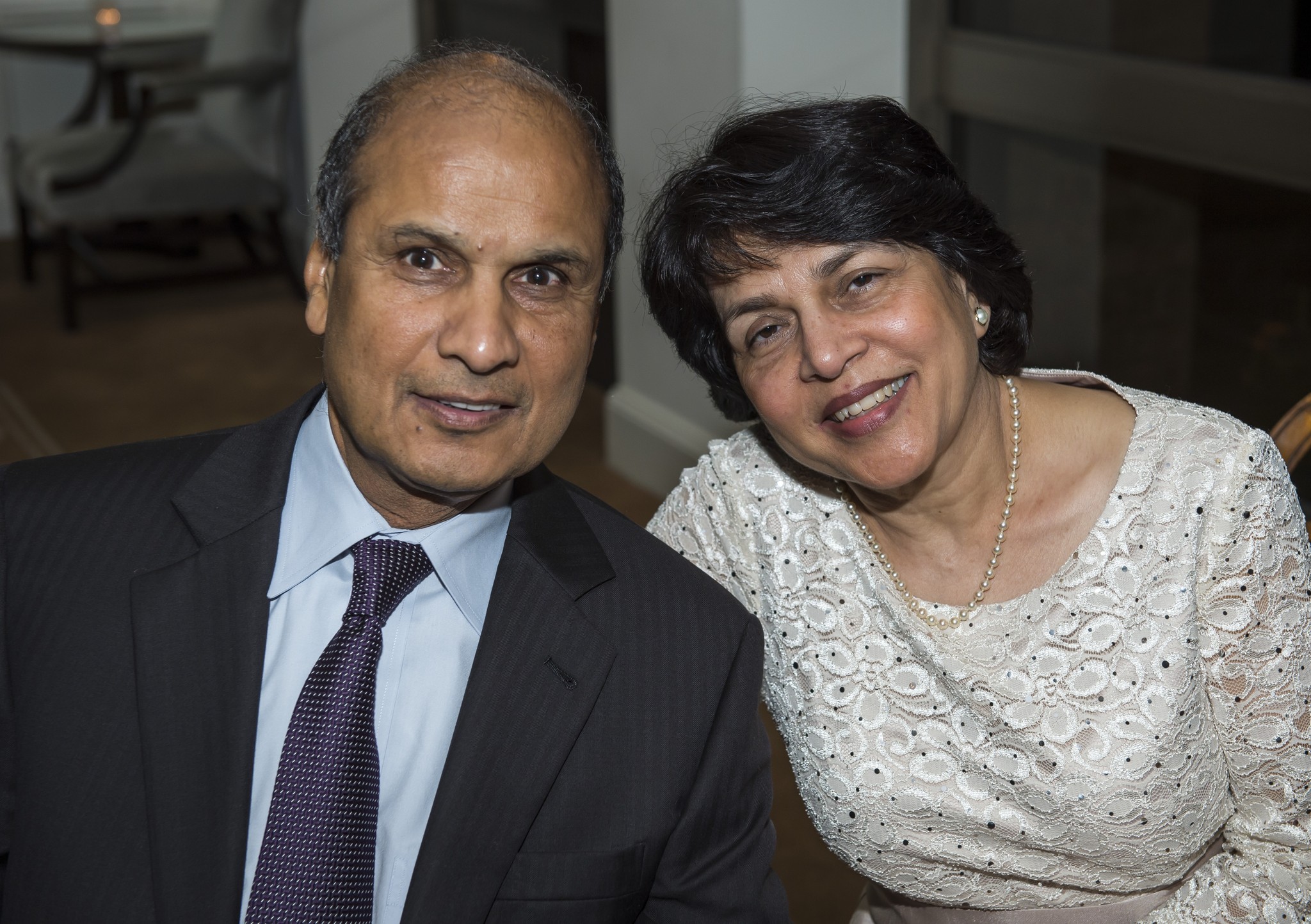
[818,370,1022,629]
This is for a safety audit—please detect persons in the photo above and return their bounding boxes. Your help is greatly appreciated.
[4,45,778,921]
[635,85,1311,924]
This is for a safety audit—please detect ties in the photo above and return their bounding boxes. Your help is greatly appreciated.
[244,534,436,924]
